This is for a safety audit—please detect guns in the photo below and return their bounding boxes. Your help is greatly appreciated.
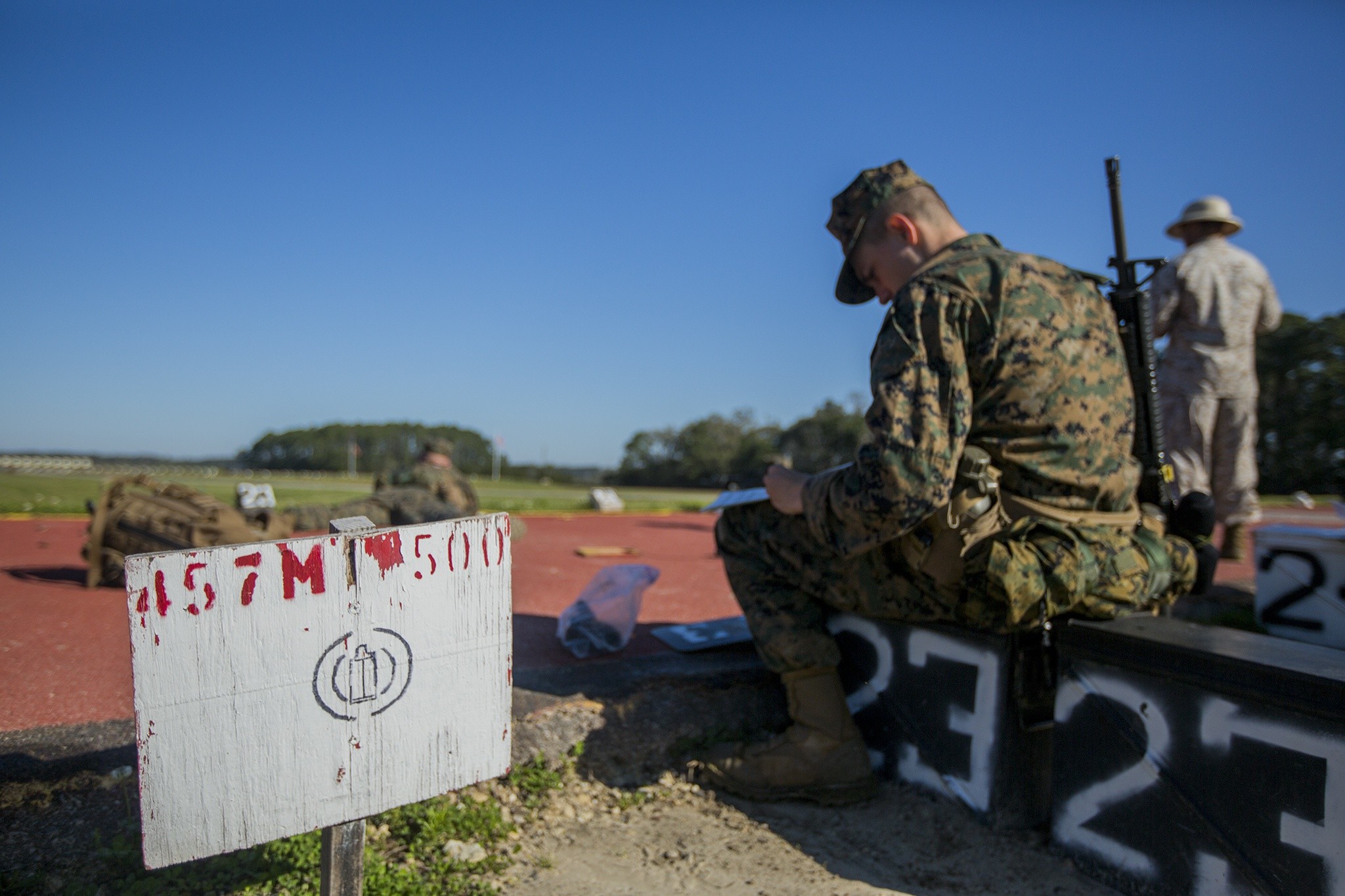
[1102,155,1234,625]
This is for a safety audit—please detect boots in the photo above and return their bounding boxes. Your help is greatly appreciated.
[1218,525,1247,559]
[702,667,874,804]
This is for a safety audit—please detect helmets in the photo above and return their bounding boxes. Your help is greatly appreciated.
[1167,196,1242,236]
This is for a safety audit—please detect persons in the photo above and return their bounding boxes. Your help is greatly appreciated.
[664,159,1139,811]
[381,437,480,517]
[1150,195,1283,565]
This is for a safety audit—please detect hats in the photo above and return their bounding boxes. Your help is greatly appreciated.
[826,158,930,304]
[425,438,452,455]
